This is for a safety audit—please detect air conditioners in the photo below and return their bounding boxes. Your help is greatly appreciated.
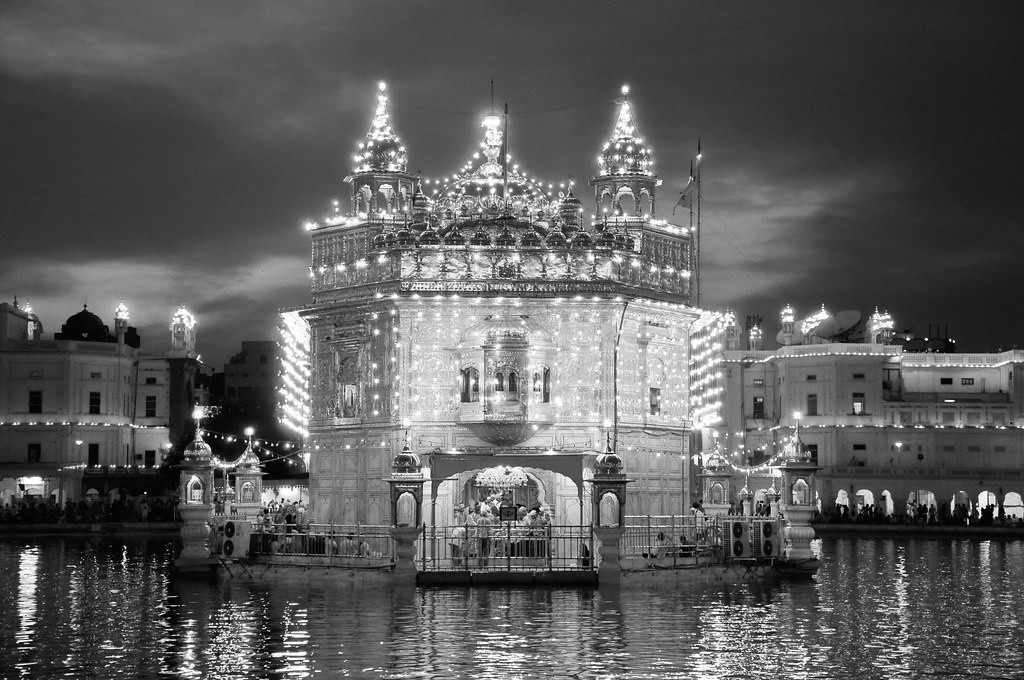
[755,520,780,558]
[722,520,751,558]
[222,519,252,559]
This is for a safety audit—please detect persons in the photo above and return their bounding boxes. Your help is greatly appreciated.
[448,496,551,570]
[816,497,1024,527]
[267,514,383,557]
[642,498,771,558]
[570,543,589,570]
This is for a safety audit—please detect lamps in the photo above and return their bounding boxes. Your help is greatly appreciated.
[475,467,528,489]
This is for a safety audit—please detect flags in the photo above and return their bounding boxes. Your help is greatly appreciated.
[673,181,691,215]
